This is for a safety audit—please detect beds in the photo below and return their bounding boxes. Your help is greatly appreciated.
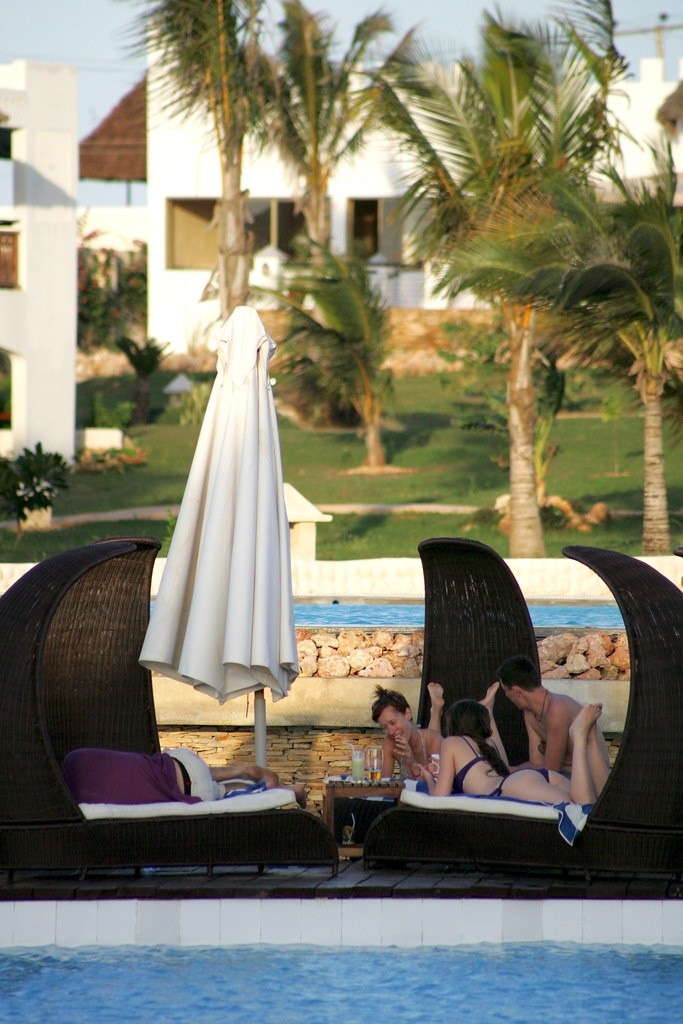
[0,537,339,886]
[362,546,683,886]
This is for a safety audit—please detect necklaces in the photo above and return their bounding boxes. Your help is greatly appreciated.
[535,689,548,721]
[418,730,427,763]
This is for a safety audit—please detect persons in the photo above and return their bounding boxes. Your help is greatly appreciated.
[372,682,511,780]
[62,747,306,809]
[497,655,608,799]
[418,699,611,804]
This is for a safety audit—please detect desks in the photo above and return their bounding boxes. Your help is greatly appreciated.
[322,780,406,857]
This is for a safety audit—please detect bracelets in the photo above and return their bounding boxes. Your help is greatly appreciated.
[412,767,421,777]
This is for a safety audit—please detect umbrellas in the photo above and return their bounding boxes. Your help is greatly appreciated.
[139,305,300,769]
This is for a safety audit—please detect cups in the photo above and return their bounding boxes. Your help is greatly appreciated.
[367,748,383,779]
[352,751,365,779]
